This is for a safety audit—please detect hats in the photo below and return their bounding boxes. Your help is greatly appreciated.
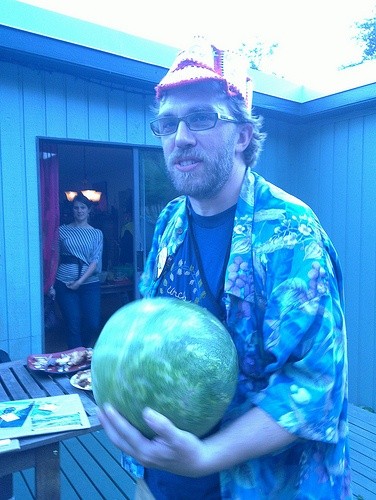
[155,39,253,111]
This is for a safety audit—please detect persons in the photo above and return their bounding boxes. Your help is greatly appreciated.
[97,35,350,500]
[49,189,103,349]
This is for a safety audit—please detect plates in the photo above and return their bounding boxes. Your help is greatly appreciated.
[27,347,90,375]
[70,368,92,391]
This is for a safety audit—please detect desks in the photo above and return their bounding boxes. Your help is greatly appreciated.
[100,280,135,294]
[0,361,103,500]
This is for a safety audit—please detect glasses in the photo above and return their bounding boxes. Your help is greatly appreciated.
[150,112,252,136]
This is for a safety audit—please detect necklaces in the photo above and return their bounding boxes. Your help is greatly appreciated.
[188,213,233,321]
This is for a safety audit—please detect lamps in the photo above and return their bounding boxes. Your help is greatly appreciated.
[63,146,102,203]
